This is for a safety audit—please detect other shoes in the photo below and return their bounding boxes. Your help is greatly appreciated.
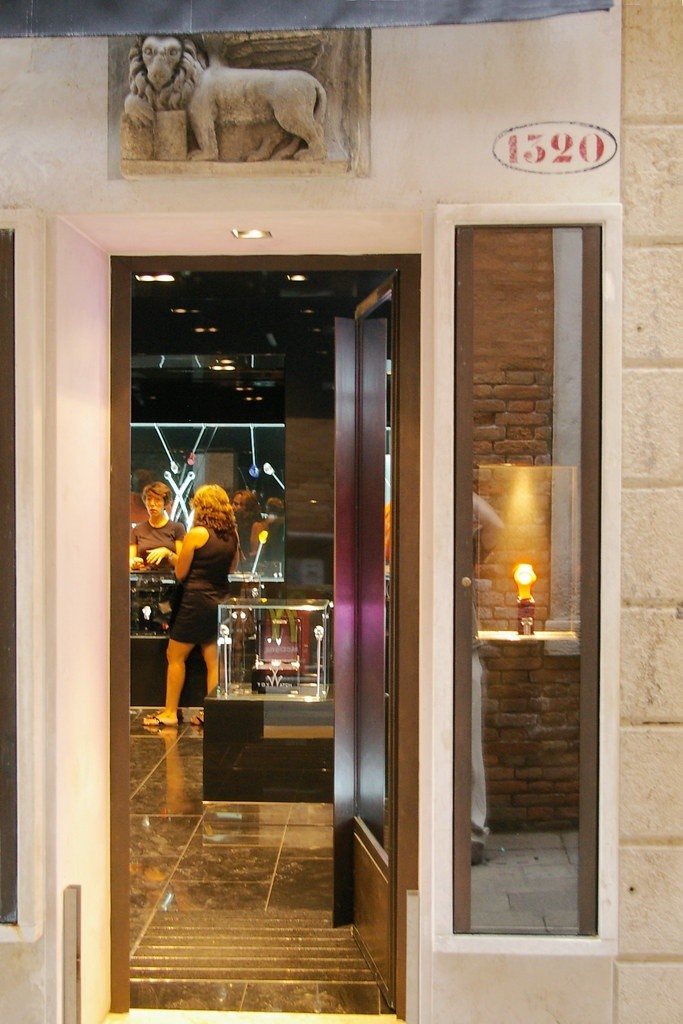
[142,710,178,726]
[191,710,204,726]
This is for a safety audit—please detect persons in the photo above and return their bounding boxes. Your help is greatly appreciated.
[232,491,285,560]
[142,484,240,728]
[471,493,505,864]
[129,481,186,571]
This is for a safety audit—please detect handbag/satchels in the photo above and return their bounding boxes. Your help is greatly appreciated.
[232,609,255,637]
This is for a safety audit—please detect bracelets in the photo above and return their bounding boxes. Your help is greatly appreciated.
[168,550,173,561]
